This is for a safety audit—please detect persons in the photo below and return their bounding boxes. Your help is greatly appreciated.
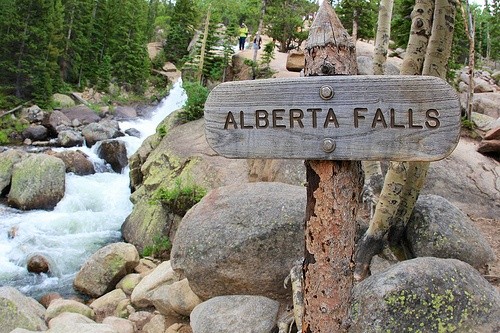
[253,32,262,50]
[237,23,250,51]
